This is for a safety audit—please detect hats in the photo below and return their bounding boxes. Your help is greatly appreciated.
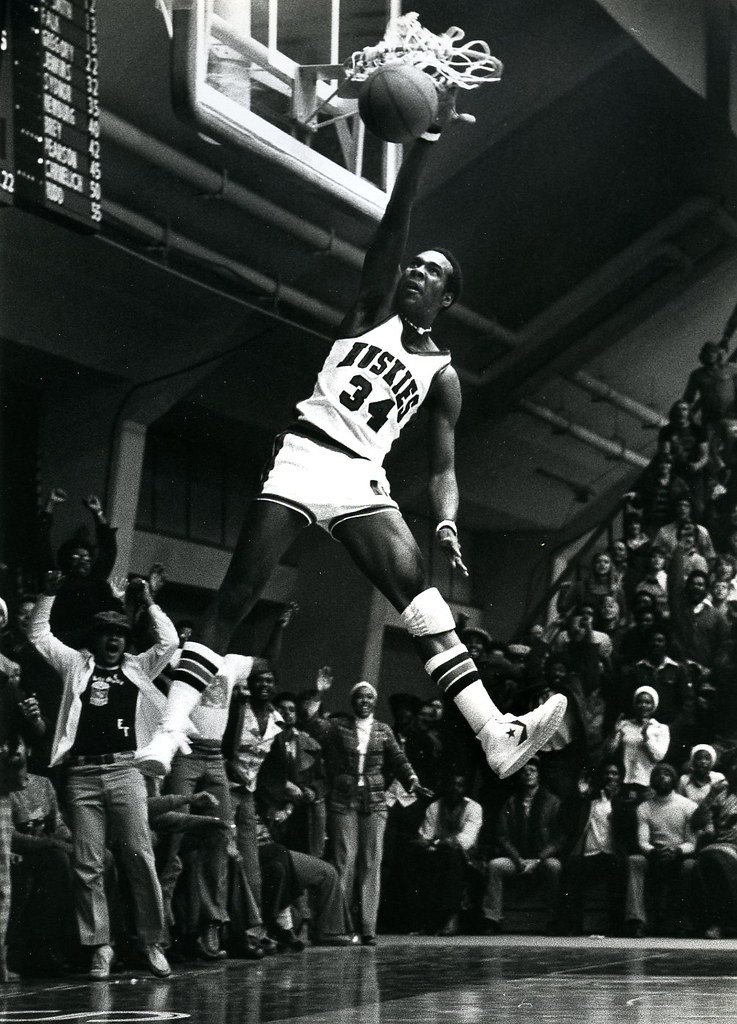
[350,681,378,700]
[690,744,716,769]
[633,686,659,713]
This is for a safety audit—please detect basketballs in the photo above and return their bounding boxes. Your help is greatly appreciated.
[356,62,440,144]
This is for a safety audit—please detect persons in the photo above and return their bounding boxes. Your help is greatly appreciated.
[1,308,737,978]
[131,80,569,778]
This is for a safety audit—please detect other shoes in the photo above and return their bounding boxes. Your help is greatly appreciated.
[226,941,264,958]
[133,939,172,977]
[310,934,353,945]
[195,943,226,959]
[88,945,114,980]
[203,920,220,952]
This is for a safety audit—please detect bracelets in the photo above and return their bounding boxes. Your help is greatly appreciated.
[418,125,442,145]
[435,519,457,539]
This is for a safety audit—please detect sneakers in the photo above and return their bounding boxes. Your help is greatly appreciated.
[476,694,568,780]
[135,730,180,776]
